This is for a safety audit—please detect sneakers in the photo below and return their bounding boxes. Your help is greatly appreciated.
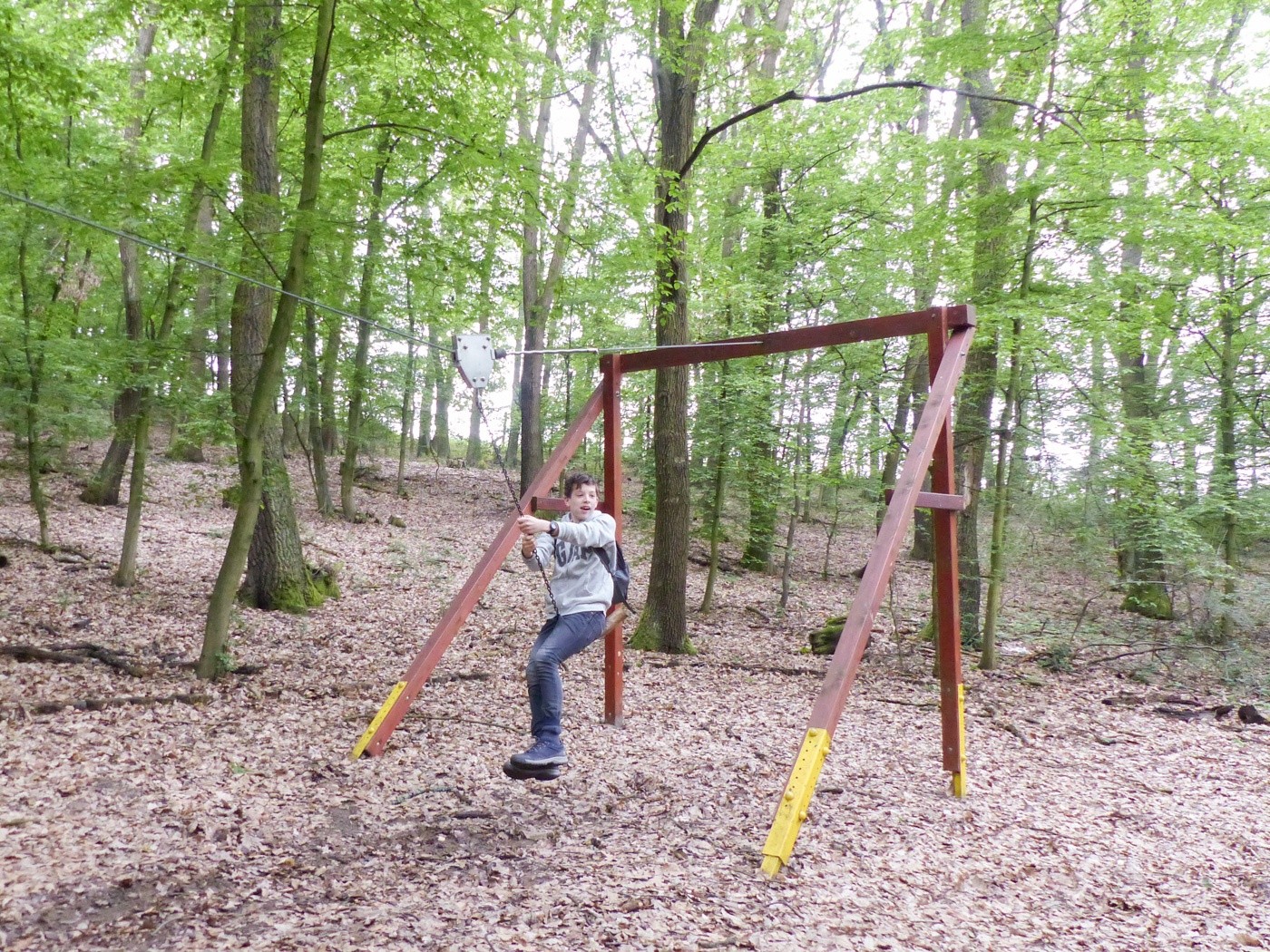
[511,739,567,768]
[502,759,562,780]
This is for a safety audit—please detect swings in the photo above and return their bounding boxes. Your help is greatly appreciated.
[449,332,630,644]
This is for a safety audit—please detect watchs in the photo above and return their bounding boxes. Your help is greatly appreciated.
[546,522,556,534]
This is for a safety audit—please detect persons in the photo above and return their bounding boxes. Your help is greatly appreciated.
[502,473,617,781]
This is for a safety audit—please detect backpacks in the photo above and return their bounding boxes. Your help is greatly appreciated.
[593,541,636,614]
